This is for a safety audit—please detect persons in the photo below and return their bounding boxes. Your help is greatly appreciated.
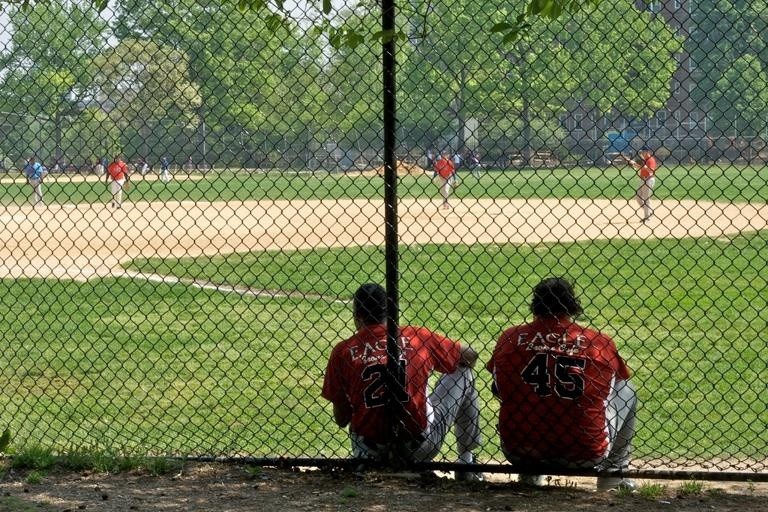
[629,146,656,223]
[25,157,44,208]
[431,150,459,210]
[106,152,131,209]
[320,283,486,482]
[0,152,192,182]
[483,277,638,495]
[425,144,481,179]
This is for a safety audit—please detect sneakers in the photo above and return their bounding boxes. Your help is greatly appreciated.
[455,460,486,481]
[518,474,544,486]
[597,478,638,490]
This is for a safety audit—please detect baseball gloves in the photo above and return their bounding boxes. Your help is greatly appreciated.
[125,182,131,190]
[635,155,645,166]
[453,180,459,187]
